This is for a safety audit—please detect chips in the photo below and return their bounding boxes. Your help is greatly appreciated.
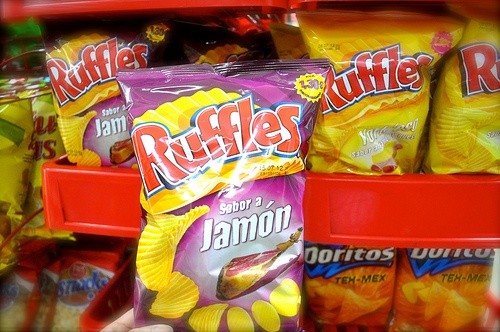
[0,2,500,331]
